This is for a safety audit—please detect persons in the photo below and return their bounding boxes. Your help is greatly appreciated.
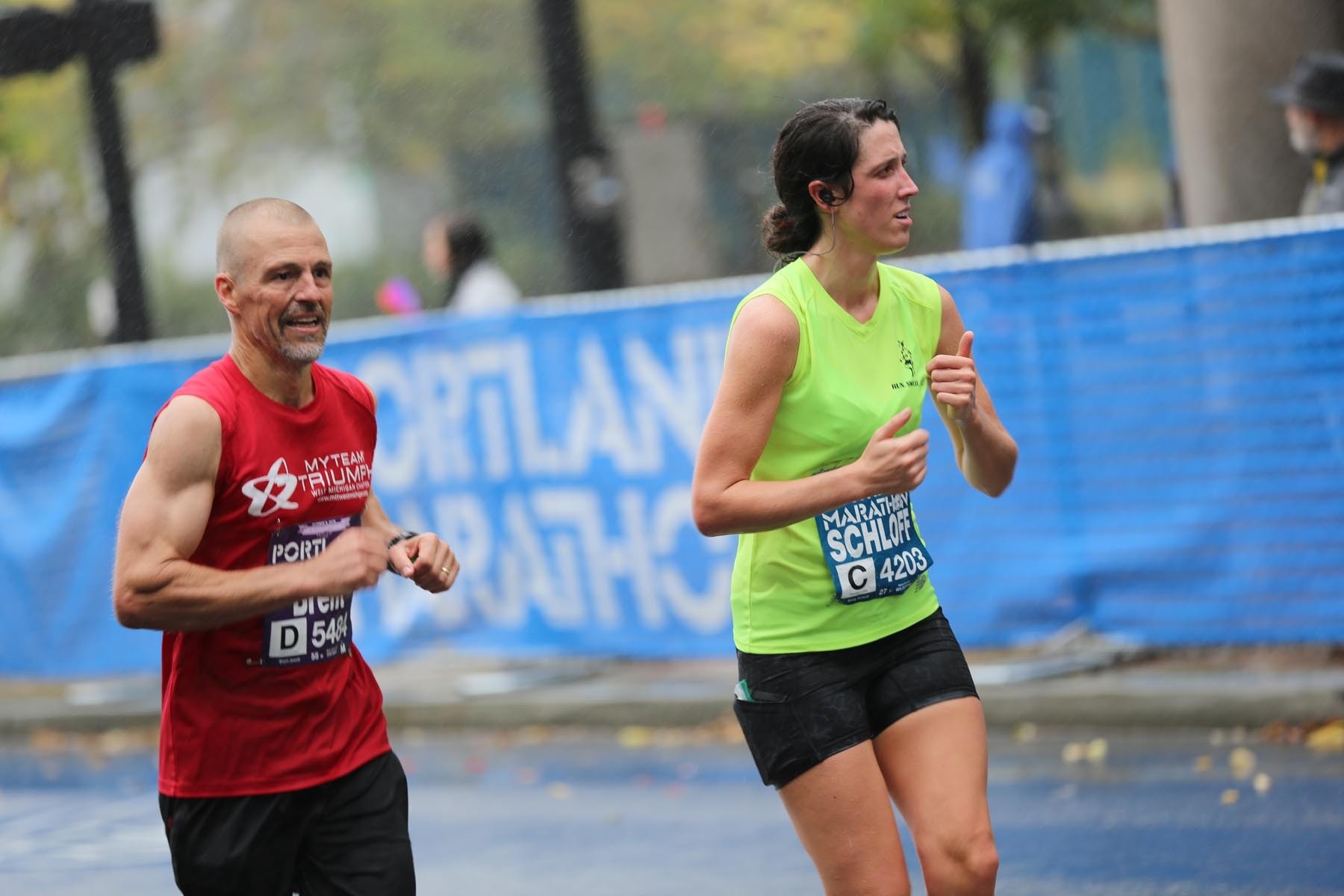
[1263,48,1344,215]
[964,98,1048,249]
[112,199,461,896]
[692,96,1019,896]
[422,208,522,310]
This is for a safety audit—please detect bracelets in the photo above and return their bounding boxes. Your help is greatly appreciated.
[385,530,420,576]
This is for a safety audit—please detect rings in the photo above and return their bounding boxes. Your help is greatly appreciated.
[441,567,449,574]
